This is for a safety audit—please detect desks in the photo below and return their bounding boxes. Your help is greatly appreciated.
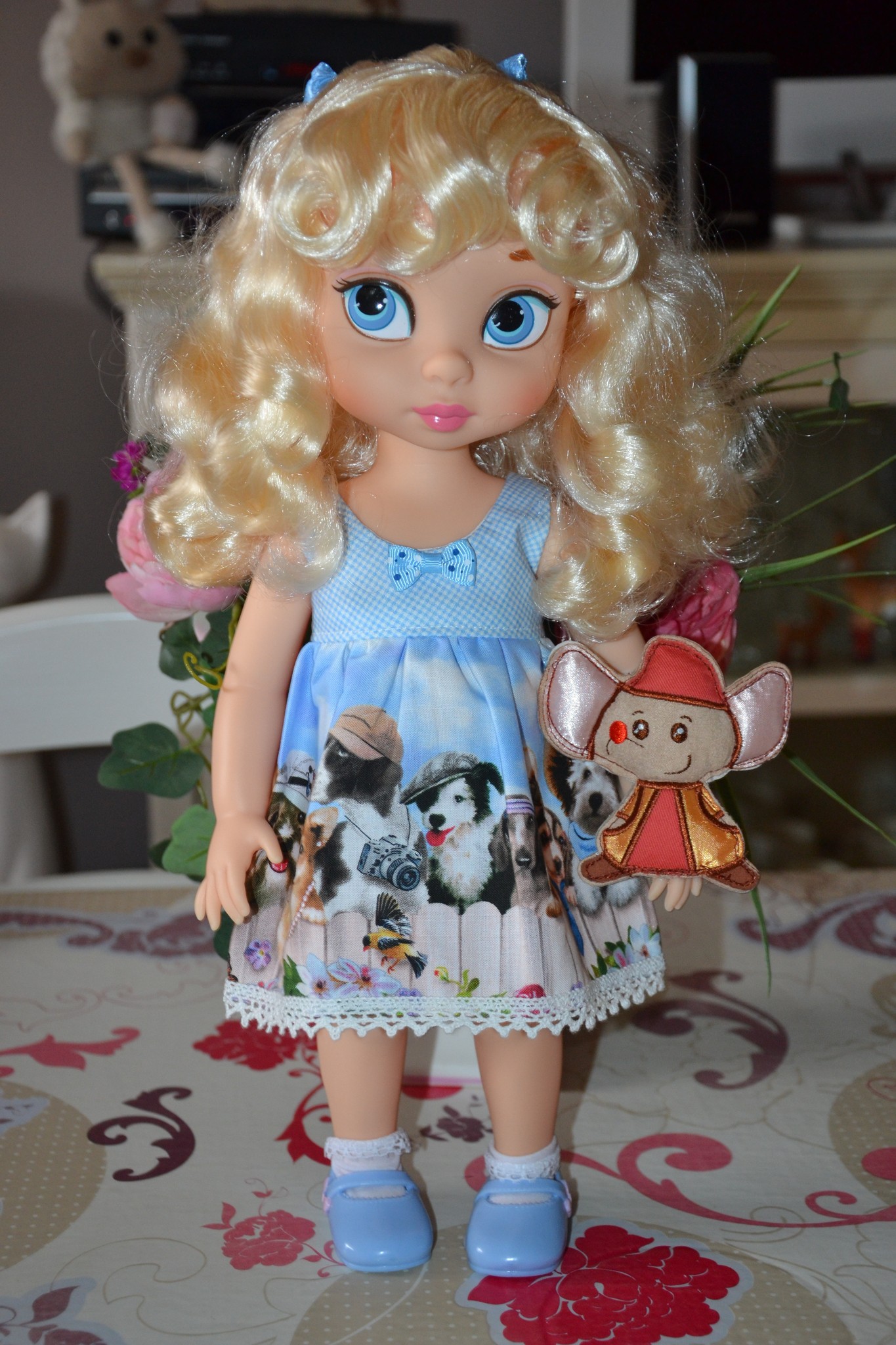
[1,865,896,1345]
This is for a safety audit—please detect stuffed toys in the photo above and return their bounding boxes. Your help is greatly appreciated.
[41,0,237,253]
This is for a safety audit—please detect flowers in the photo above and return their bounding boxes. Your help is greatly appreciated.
[97,267,894,993]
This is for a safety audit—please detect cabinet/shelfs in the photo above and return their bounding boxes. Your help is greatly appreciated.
[94,243,896,870]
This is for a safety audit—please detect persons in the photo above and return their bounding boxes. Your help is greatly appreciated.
[143,45,751,1278]
